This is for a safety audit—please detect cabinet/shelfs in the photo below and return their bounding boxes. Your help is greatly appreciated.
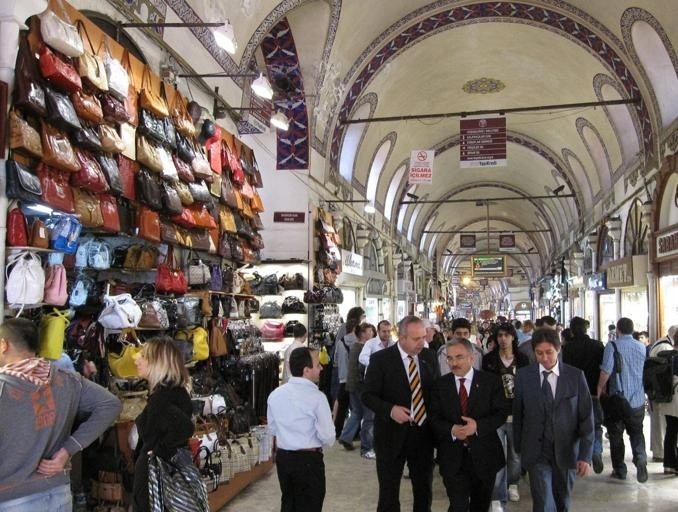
[100,283,276,512]
[244,258,308,358]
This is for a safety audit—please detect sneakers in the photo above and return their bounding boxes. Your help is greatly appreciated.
[361,450,375,459]
[637,466,648,482]
[611,470,626,479]
[508,484,520,502]
[339,438,353,450]
[491,499,503,512]
[592,452,603,473]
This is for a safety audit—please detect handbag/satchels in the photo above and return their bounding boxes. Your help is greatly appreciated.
[601,394,633,424]
[642,357,673,403]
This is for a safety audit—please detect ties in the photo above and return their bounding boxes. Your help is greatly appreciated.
[407,353,428,427]
[542,371,554,441]
[458,378,468,416]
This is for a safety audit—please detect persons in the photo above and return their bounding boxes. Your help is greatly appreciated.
[326,303,678,483]
[280,321,310,384]
[513,329,597,512]
[130,336,197,512]
[431,335,508,512]
[484,323,526,512]
[1,315,124,512]
[359,314,441,512]
[255,346,338,512]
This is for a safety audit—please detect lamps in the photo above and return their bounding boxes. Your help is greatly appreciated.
[210,87,290,131]
[317,199,376,213]
[116,15,237,56]
[178,69,273,100]
